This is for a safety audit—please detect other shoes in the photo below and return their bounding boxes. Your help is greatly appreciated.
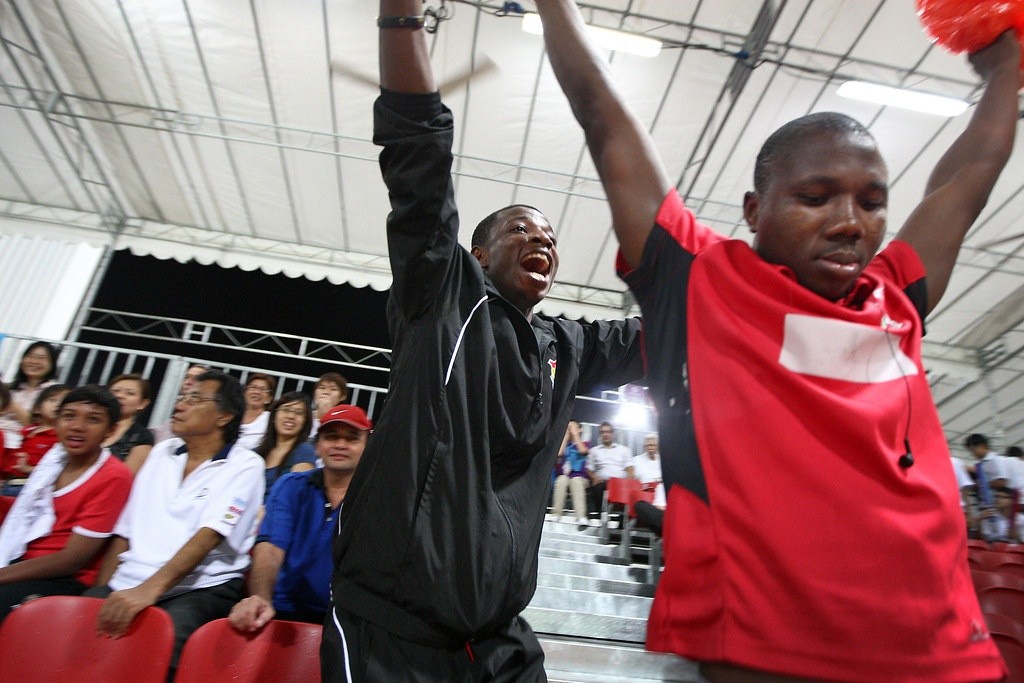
[548,514,558,522]
[579,517,589,526]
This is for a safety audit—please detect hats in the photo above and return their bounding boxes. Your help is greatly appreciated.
[317,405,372,438]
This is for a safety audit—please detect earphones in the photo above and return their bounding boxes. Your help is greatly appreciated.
[899,438,914,467]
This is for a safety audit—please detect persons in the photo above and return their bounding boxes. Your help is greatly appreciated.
[323,0,650,683]
[539,0,1022,683]
[939,432,1024,547]
[550,419,666,538]
[0,341,369,639]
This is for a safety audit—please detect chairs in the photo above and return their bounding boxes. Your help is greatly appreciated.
[599,478,1023,682]
[0,495,323,683]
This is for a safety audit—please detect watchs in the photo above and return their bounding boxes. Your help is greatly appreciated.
[378,16,425,29]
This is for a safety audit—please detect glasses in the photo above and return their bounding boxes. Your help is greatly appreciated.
[245,384,272,396]
[175,391,221,407]
[274,407,309,421]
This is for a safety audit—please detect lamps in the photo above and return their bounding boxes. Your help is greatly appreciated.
[521,11,667,58]
[836,80,968,118]
